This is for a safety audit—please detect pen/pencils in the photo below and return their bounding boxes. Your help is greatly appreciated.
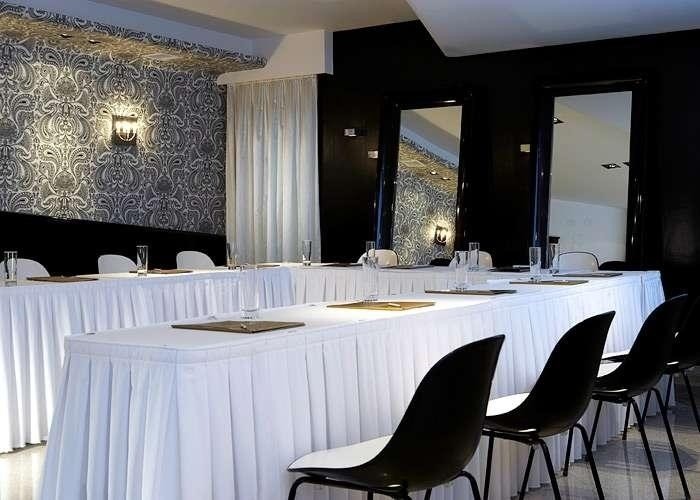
[240,322,253,333]
[388,302,400,307]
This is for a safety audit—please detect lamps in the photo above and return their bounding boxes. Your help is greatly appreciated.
[435,225,448,245]
[111,113,137,146]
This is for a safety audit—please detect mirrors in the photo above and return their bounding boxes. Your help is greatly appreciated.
[387,94,467,266]
[542,80,638,271]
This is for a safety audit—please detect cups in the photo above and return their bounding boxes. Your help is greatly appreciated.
[301,240,312,266]
[136,244,148,276]
[528,247,541,281]
[237,264,261,331]
[468,241,480,270]
[363,257,379,301]
[3,250,17,286]
[548,242,560,276]
[366,240,376,263]
[224,242,240,271]
[454,248,469,289]
